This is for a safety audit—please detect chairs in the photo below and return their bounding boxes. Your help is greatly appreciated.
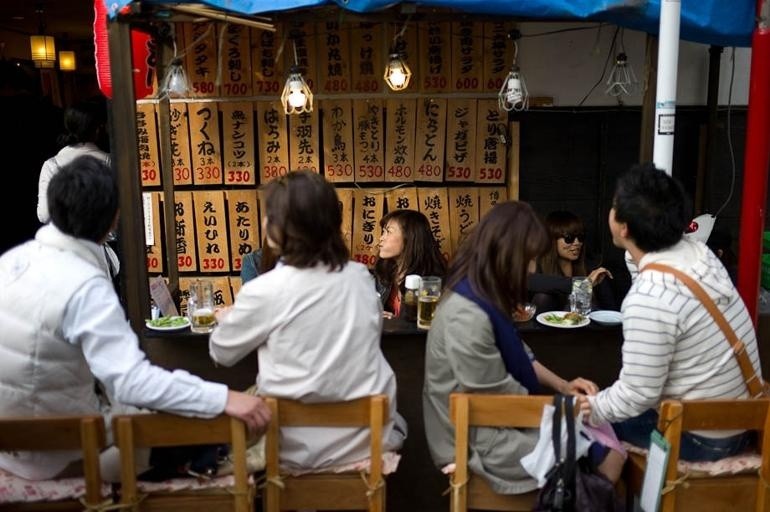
[1,416,107,510]
[627,397,769,510]
[260,396,391,511]
[448,392,589,511]
[108,409,259,510]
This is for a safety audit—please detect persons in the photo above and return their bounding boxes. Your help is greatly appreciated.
[237,228,282,287]
[0,153,275,485]
[536,210,623,310]
[522,263,616,295]
[365,209,453,311]
[423,199,628,494]
[575,159,764,462]
[206,168,410,473]
[36,101,125,311]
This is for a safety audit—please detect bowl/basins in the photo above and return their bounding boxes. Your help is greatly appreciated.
[511,303,536,321]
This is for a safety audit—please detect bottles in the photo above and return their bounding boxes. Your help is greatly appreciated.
[403,274,423,323]
[151,305,160,320]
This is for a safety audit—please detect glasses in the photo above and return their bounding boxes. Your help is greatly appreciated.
[558,233,585,244]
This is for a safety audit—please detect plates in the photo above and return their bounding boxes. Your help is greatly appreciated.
[147,314,191,331]
[590,309,623,325]
[535,309,590,329]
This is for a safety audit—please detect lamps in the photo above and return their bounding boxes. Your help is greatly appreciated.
[161,21,193,94]
[382,5,421,92]
[280,21,315,114]
[57,30,77,72]
[603,22,641,97]
[496,27,532,113]
[28,0,58,70]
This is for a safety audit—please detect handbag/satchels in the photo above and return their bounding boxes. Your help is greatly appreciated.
[532,461,614,512]
[99,385,266,480]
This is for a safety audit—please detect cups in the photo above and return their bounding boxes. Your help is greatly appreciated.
[417,278,442,329]
[187,279,214,332]
[571,276,592,313]
